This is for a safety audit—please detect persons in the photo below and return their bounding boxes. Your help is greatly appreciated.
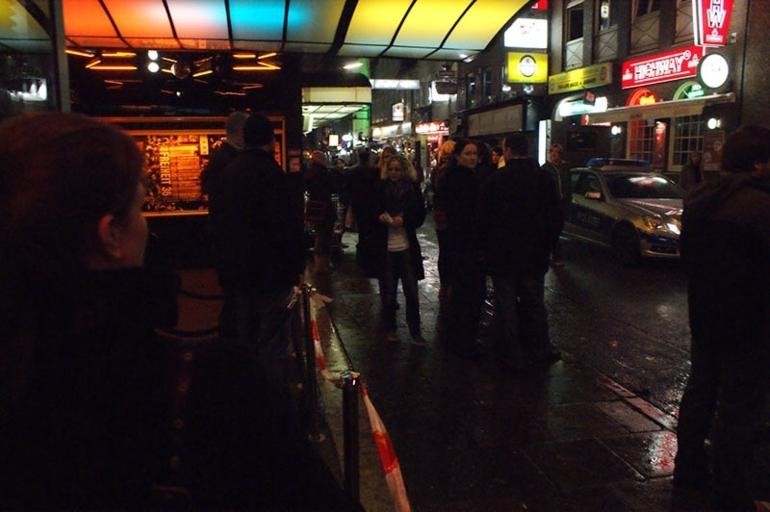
[0,111,366,512]
[680,152,704,194]
[210,113,304,379]
[201,111,248,339]
[672,126,770,511]
[304,126,573,368]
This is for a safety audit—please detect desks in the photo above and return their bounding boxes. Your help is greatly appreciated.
[141,208,210,270]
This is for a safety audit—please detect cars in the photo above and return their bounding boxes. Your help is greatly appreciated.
[559,157,691,266]
